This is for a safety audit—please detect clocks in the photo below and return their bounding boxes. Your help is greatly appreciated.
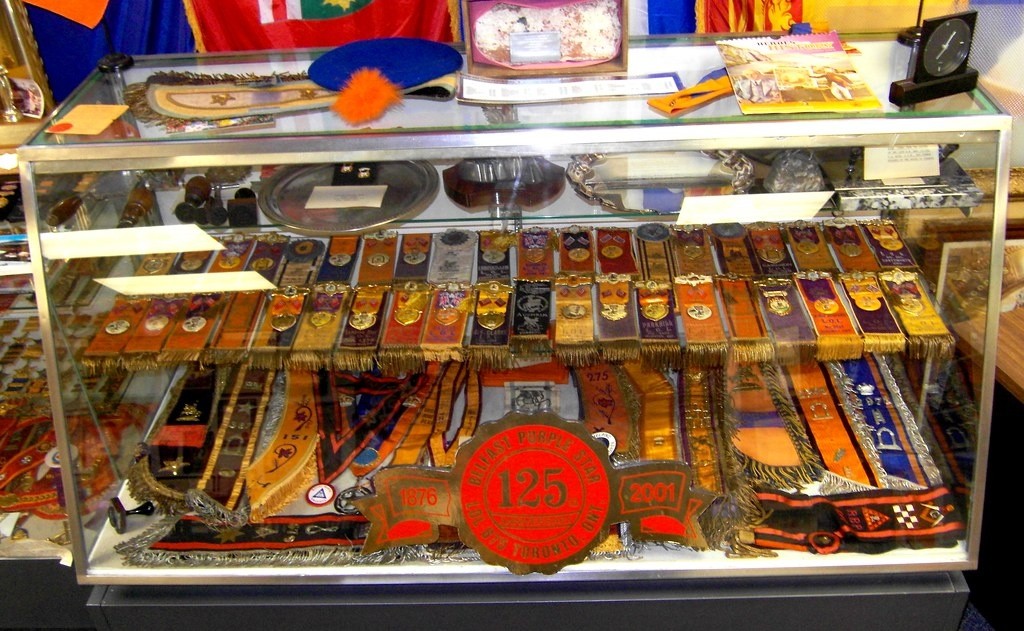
[889,9,979,106]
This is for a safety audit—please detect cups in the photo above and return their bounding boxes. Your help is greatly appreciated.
[99,52,137,109]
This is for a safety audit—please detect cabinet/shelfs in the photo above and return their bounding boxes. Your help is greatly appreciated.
[17,32,1013,584]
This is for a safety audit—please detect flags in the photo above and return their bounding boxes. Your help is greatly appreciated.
[20,0,803,105]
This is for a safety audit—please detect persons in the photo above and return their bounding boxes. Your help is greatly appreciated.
[734,69,778,105]
[809,68,854,102]
